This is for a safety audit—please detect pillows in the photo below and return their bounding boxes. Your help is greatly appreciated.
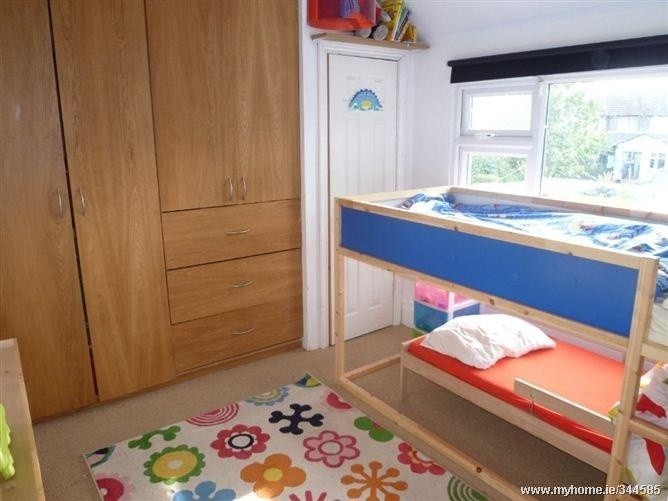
[420,314,556,370]
[607,363,668,500]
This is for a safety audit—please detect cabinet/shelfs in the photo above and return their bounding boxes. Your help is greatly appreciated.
[162,199,303,374]
[145,0,301,212]
[0,0,176,424]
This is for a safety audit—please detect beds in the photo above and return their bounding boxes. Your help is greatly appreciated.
[333,185,668,501]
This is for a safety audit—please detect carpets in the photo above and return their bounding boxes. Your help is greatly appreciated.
[83,372,487,501]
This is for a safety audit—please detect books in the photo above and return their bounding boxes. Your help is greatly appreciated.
[379,0,412,42]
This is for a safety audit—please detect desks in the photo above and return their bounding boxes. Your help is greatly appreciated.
[0,337,46,501]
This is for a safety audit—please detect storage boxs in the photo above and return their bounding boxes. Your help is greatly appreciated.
[413,280,481,341]
